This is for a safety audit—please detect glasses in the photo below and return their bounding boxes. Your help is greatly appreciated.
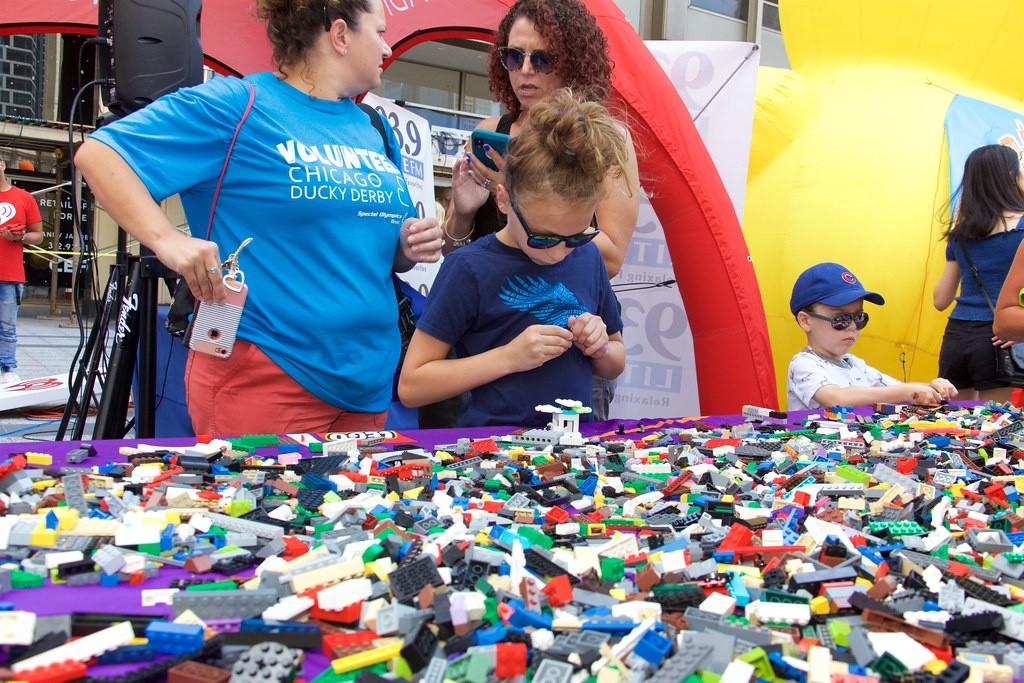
[509,190,601,251]
[498,46,559,75]
[805,311,869,331]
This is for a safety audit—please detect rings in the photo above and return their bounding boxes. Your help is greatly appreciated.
[484,179,490,185]
[209,267,220,273]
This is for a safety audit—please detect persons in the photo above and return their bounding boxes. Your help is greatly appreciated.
[933,145,1024,405]
[1,155,43,386]
[787,262,959,411]
[439,1,639,421]
[397,89,625,427]
[992,238,1024,349]
[74,0,443,439]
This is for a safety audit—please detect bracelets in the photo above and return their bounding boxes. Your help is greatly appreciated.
[19,235,24,242]
[444,214,476,247]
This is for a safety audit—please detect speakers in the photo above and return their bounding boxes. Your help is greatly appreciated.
[98,0,202,119]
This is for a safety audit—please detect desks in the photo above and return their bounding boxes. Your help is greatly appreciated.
[0,403,1023,683]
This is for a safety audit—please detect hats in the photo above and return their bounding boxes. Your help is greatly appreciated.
[790,263,885,315]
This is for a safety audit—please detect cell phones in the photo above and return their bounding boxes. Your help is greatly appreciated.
[189,280,248,359]
[471,129,511,172]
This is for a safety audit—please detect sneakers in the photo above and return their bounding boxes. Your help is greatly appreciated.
[0,372,21,385]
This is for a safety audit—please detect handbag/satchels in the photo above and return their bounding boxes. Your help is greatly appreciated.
[164,277,195,338]
[994,342,1024,385]
[391,294,417,403]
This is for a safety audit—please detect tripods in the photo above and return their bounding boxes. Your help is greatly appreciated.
[56,225,176,440]
